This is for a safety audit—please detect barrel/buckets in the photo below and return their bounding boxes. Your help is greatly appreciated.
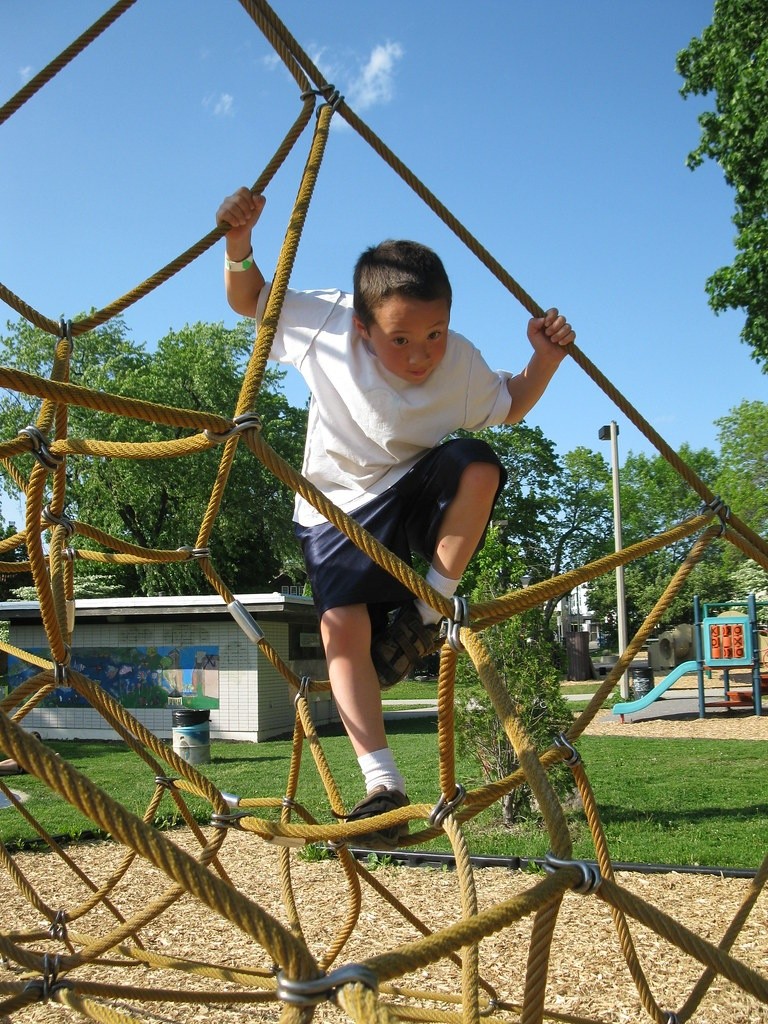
[632,667,655,701]
[172,709,211,764]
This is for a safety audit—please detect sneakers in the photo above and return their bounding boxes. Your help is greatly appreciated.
[367,597,451,692]
[327,784,410,852]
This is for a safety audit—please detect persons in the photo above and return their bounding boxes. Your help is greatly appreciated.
[214,183,578,853]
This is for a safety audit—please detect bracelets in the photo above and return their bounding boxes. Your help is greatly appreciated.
[223,246,255,273]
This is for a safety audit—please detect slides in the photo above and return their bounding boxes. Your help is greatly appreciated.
[611,660,697,715]
